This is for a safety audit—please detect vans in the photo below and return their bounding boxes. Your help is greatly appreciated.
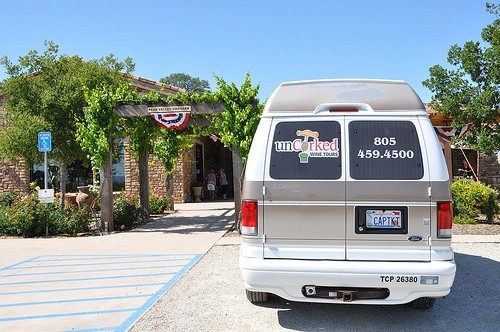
[238,78,457,310]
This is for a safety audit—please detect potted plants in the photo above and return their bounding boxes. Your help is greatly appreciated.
[191,181,204,202]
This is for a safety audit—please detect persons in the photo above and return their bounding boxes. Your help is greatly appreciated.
[458,160,472,175]
[217,168,228,200]
[205,169,216,200]
